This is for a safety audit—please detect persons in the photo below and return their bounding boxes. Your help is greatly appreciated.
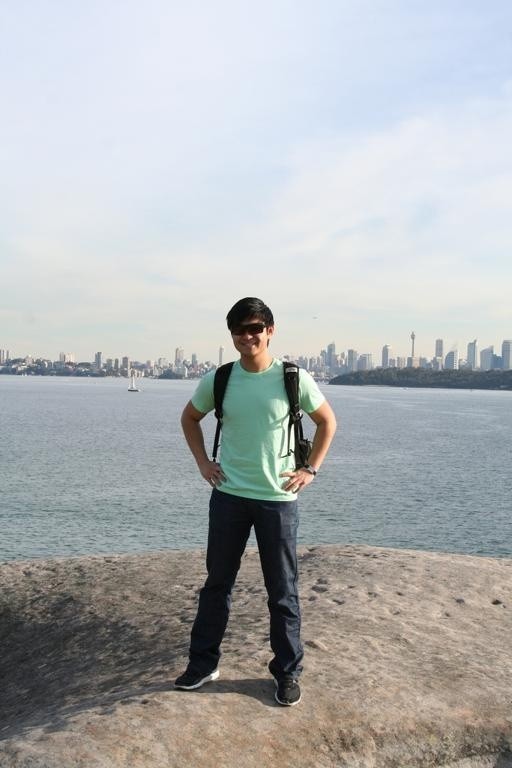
[171,296,339,707]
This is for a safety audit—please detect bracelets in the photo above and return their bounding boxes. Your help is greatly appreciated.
[303,463,317,477]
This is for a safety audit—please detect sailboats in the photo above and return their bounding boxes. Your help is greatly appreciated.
[127,375,141,392]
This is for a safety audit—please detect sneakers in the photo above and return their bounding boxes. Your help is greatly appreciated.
[273,671,302,706]
[174,661,220,690]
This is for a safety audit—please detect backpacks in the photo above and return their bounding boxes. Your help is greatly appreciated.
[213,360,312,472]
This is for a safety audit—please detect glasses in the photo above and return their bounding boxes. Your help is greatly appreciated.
[231,322,269,335]
